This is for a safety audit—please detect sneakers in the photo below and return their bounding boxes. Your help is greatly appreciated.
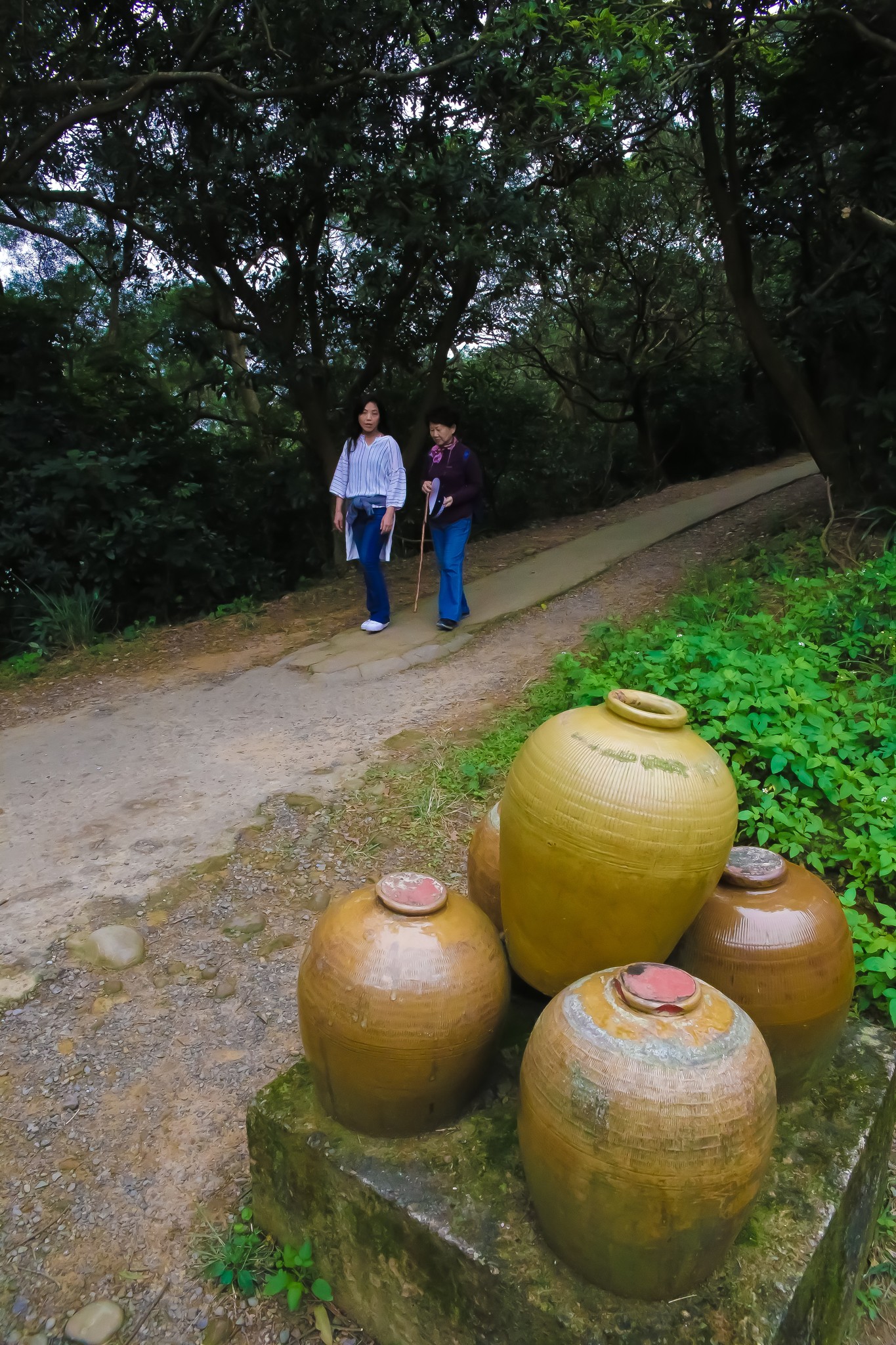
[436,619,457,629]
[366,621,390,632]
[460,612,469,617]
[361,619,371,630]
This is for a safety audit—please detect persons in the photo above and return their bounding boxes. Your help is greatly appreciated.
[417,407,482,629]
[329,395,406,632]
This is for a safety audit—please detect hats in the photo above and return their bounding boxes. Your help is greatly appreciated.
[429,478,446,518]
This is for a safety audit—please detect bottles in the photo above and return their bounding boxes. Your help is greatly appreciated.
[670,845,857,1101]
[296,871,516,1137]
[502,687,741,998]
[467,798,502,931]
[515,960,782,1300]
